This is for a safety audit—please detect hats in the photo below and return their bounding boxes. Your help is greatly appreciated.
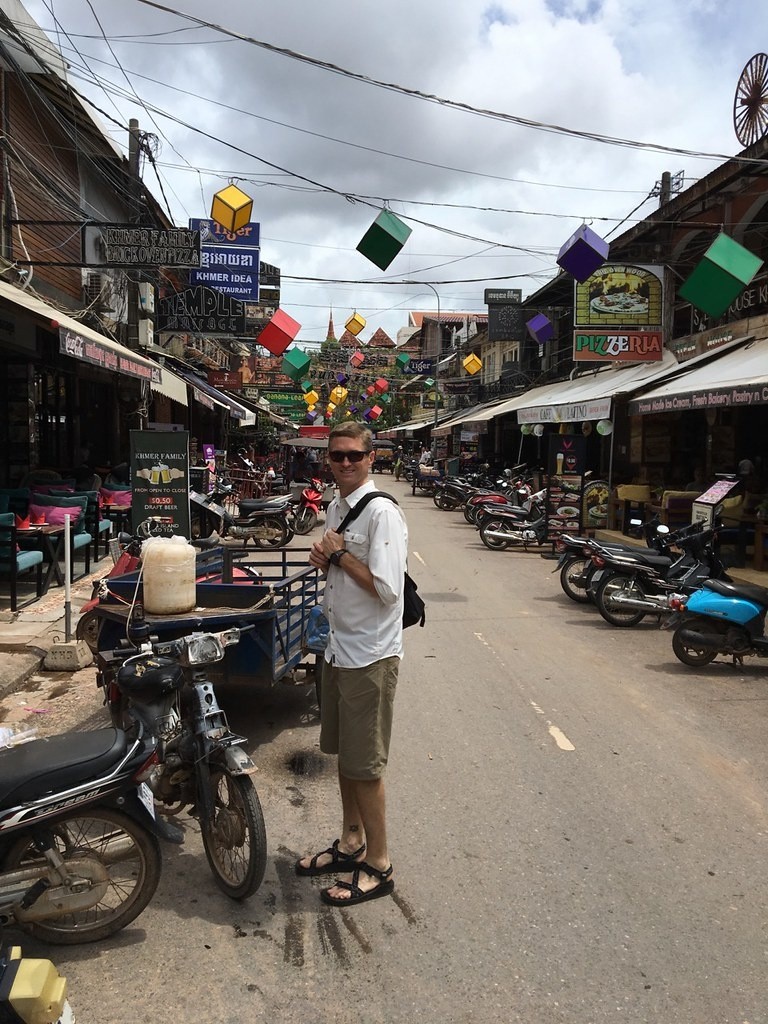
[398,446,403,449]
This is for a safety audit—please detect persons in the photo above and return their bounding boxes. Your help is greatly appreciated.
[419,446,432,464]
[393,445,404,482]
[293,423,409,905]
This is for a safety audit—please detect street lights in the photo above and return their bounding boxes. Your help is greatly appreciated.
[403,280,440,460]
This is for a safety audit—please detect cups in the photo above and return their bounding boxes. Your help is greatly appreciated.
[160,467,174,484]
[557,454,563,474]
[148,469,161,485]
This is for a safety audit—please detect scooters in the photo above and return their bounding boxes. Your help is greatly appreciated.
[659,576,768,667]
[75,517,263,654]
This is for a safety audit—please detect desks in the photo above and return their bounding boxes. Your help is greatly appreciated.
[631,499,662,539]
[721,515,768,571]
[100,502,117,541]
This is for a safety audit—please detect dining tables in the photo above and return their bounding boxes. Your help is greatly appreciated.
[17,524,74,595]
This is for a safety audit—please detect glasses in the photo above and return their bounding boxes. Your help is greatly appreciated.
[327,449,371,464]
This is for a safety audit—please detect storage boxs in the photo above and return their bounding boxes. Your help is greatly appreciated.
[420,468,431,476]
[431,470,439,477]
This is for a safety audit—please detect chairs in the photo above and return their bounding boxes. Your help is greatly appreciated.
[0,493,10,512]
[30,476,77,494]
[47,488,111,561]
[28,493,92,583]
[98,481,132,538]
[0,487,30,511]
[0,512,45,612]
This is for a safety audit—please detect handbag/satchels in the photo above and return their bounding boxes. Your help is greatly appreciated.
[400,572,426,630]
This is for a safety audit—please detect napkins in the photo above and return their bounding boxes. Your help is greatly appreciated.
[16,513,31,528]
[32,510,46,523]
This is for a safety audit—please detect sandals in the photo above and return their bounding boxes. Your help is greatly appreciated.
[296,839,366,876]
[319,861,397,906]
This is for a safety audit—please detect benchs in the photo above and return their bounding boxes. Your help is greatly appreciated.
[646,490,701,532]
[610,484,656,535]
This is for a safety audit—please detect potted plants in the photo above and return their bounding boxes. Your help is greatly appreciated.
[754,499,768,521]
[655,486,666,502]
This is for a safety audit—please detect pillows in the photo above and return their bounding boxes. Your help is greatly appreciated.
[31,483,75,495]
[99,487,132,508]
[30,504,83,525]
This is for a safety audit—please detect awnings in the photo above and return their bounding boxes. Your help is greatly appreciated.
[377,335,768,440]
[0,279,294,430]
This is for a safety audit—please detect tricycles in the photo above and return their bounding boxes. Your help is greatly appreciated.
[411,462,443,495]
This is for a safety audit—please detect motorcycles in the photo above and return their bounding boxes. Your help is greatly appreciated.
[107,640,267,899]
[432,470,547,551]
[0,719,186,945]
[191,468,298,548]
[403,460,418,482]
[281,438,336,515]
[551,512,733,627]
[292,477,326,535]
[371,440,395,474]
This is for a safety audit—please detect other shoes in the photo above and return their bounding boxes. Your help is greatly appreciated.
[395,479,397,482]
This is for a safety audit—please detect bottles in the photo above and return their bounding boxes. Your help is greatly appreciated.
[142,537,197,614]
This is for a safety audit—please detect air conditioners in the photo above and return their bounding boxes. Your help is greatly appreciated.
[85,272,117,314]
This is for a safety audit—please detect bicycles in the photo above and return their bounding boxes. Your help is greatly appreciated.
[141,516,263,585]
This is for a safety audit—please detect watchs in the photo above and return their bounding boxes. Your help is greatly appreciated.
[330,549,350,567]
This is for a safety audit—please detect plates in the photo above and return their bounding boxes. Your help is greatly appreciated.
[567,522,580,527]
[588,504,608,518]
[17,527,37,530]
[549,519,563,527]
[29,522,50,525]
[557,506,580,517]
[103,503,117,505]
[590,294,649,314]
